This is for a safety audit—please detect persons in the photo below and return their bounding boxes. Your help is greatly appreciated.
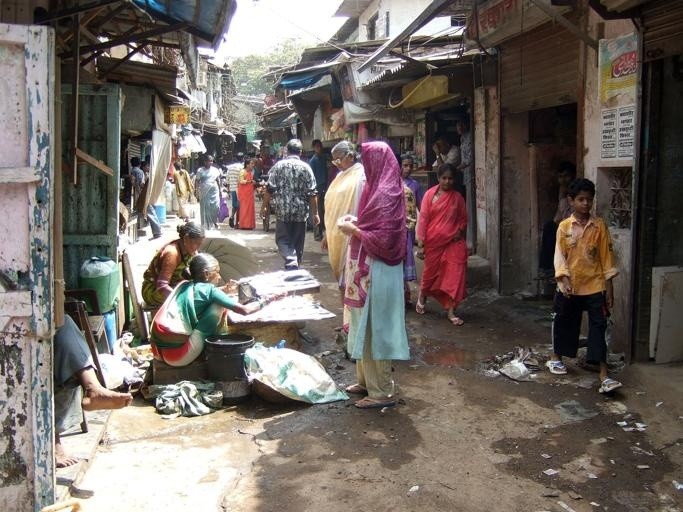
[140,220,206,307]
[148,251,289,368]
[455,117,477,256]
[50,313,134,470]
[542,174,624,395]
[125,137,329,271]
[335,140,411,409]
[319,138,369,280]
[412,163,470,327]
[429,132,460,169]
[397,151,421,307]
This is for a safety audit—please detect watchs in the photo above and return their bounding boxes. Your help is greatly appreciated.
[435,155,441,159]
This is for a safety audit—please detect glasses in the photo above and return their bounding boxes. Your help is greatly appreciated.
[331,152,349,166]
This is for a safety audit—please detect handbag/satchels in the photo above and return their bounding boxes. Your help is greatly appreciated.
[219,198,229,221]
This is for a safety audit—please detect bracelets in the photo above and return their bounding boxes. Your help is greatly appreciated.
[257,294,279,309]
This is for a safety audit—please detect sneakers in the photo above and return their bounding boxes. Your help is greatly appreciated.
[230,218,234,228]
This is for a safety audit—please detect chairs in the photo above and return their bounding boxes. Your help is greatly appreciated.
[64,288,111,389]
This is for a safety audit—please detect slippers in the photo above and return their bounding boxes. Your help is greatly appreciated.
[355,396,396,409]
[450,317,464,326]
[547,359,568,374]
[417,294,427,316]
[599,379,621,393]
[346,383,367,395]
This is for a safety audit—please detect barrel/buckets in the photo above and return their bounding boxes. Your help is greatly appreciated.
[204,333,255,382]
[98,311,117,355]
[154,204,166,224]
